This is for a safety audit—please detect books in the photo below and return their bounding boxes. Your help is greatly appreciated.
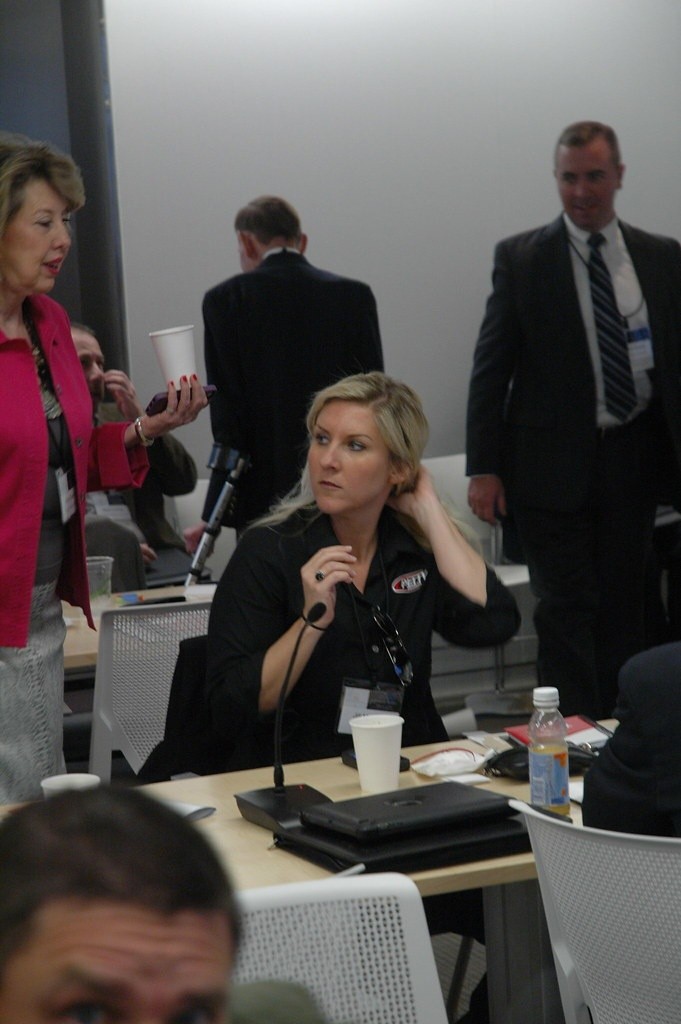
[505,715,610,747]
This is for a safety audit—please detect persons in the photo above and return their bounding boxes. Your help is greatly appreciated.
[0,785,242,1024]
[464,121,681,717]
[183,194,385,555]
[69,324,199,566]
[581,639,681,836]
[0,135,208,800]
[198,371,520,773]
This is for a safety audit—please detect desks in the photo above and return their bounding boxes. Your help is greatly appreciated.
[0,719,625,1024]
[87,601,214,782]
[62,584,217,675]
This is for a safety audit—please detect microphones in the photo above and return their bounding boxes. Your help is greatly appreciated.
[233,602,335,838]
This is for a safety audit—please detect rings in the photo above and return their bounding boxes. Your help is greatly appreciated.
[166,408,174,415]
[315,571,324,582]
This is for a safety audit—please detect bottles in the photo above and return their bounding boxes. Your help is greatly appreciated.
[526,686,570,817]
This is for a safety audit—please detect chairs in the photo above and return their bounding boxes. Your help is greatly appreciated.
[511,799,679,1022]
[224,874,446,1024]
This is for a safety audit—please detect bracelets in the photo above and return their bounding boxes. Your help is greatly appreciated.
[134,417,155,446]
[301,614,327,631]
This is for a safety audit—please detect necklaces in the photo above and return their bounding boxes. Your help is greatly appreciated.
[24,318,65,421]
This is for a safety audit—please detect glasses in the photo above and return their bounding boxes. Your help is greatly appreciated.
[372,608,413,688]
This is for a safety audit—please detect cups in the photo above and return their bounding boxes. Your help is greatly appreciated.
[78,556,115,621]
[148,326,197,391]
[349,715,405,794]
[441,707,477,737]
[41,774,101,799]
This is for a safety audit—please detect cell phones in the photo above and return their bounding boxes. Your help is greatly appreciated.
[145,386,219,418]
[342,750,411,774]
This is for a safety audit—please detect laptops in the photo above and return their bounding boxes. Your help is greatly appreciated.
[298,780,521,842]
[272,803,574,876]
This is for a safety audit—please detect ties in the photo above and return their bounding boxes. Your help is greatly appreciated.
[586,232,637,424]
